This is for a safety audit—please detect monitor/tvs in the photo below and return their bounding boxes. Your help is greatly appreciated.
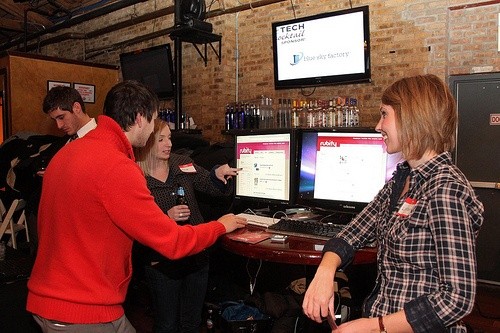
[232,129,294,216]
[271,5,371,90]
[295,127,407,223]
[120,43,176,99]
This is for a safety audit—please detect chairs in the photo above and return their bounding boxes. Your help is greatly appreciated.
[0,197,31,250]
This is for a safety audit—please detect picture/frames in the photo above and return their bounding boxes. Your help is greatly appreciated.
[46,80,71,96]
[73,82,95,104]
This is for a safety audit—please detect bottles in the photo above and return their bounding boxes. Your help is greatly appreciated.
[175,186,190,224]
[225,95,360,130]
[158,108,190,133]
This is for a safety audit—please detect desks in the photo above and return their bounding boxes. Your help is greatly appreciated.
[218,204,381,291]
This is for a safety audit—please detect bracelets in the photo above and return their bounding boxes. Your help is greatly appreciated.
[379,315,387,333]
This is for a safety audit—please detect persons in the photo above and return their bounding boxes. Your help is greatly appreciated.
[36,85,98,177]
[301,74,484,333]
[133,118,243,333]
[25,80,248,333]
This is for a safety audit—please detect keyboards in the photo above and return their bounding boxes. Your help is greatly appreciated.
[235,213,280,227]
[266,217,377,247]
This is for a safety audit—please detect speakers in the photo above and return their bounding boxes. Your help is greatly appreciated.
[175,0,206,28]
[448,71,500,293]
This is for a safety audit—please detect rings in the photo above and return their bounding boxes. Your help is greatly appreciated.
[180,213,182,217]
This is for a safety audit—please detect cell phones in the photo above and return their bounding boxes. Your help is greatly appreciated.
[271,235,288,243]
